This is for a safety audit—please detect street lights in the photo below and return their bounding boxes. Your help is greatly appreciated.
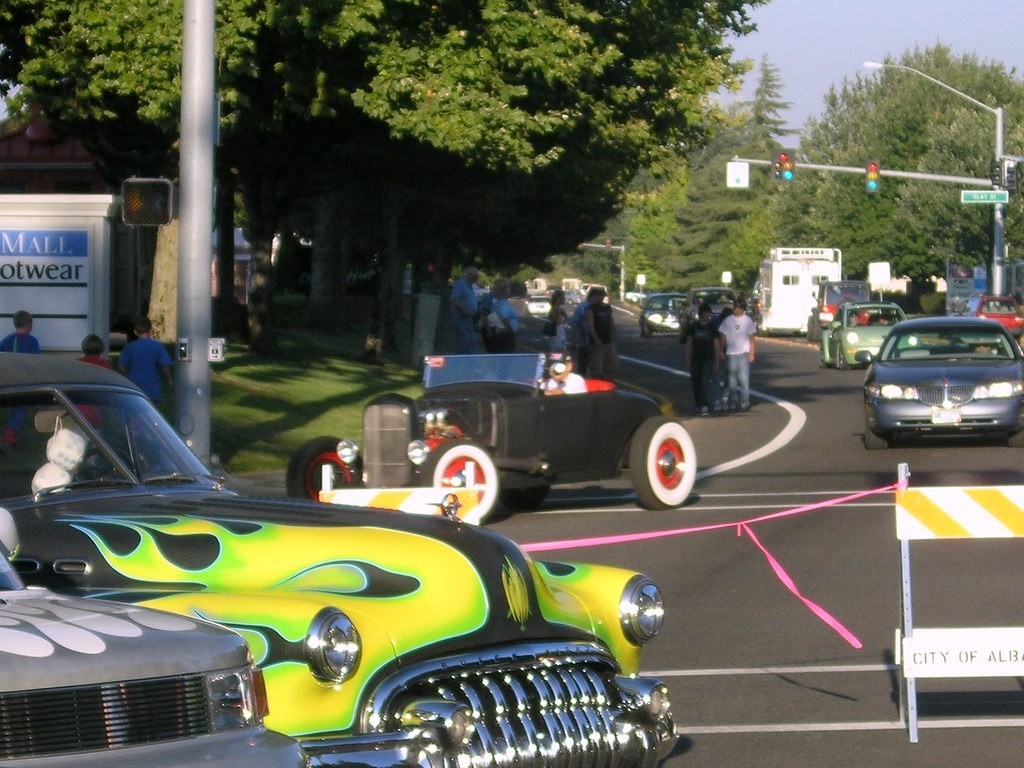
[861,59,1005,310]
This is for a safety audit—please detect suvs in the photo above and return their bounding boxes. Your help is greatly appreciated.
[677,284,744,343]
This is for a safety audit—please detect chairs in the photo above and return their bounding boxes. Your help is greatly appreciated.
[585,380,615,394]
[897,348,929,358]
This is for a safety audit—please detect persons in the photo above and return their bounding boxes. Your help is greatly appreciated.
[849,310,864,326]
[57,334,111,467]
[718,297,757,411]
[976,342,991,353]
[478,277,519,354]
[545,289,569,351]
[449,266,481,354]
[0,309,41,444]
[877,312,891,326]
[539,347,587,395]
[118,316,176,436]
[571,286,621,379]
[685,304,721,416]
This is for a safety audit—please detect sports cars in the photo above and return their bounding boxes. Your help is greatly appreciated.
[283,342,699,524]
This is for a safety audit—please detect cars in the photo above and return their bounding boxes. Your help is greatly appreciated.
[953,292,1024,341]
[522,279,610,317]
[625,288,656,304]
[820,299,921,372]
[853,313,1024,453]
[1,348,682,768]
[639,292,686,339]
[1,505,313,768]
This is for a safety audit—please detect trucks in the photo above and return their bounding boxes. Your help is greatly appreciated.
[804,280,880,343]
[749,245,843,335]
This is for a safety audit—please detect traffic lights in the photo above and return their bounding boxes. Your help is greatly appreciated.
[991,159,1002,191]
[1006,164,1020,193]
[119,175,176,227]
[770,148,795,183]
[865,159,881,194]
[605,239,611,250]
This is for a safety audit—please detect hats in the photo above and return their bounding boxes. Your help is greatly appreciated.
[466,266,480,280]
[547,348,574,363]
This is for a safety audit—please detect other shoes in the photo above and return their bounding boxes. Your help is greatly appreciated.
[741,406,749,413]
[713,400,723,411]
[730,408,739,413]
[700,405,711,415]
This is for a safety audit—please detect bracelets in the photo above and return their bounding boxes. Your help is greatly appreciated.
[714,364,719,368]
